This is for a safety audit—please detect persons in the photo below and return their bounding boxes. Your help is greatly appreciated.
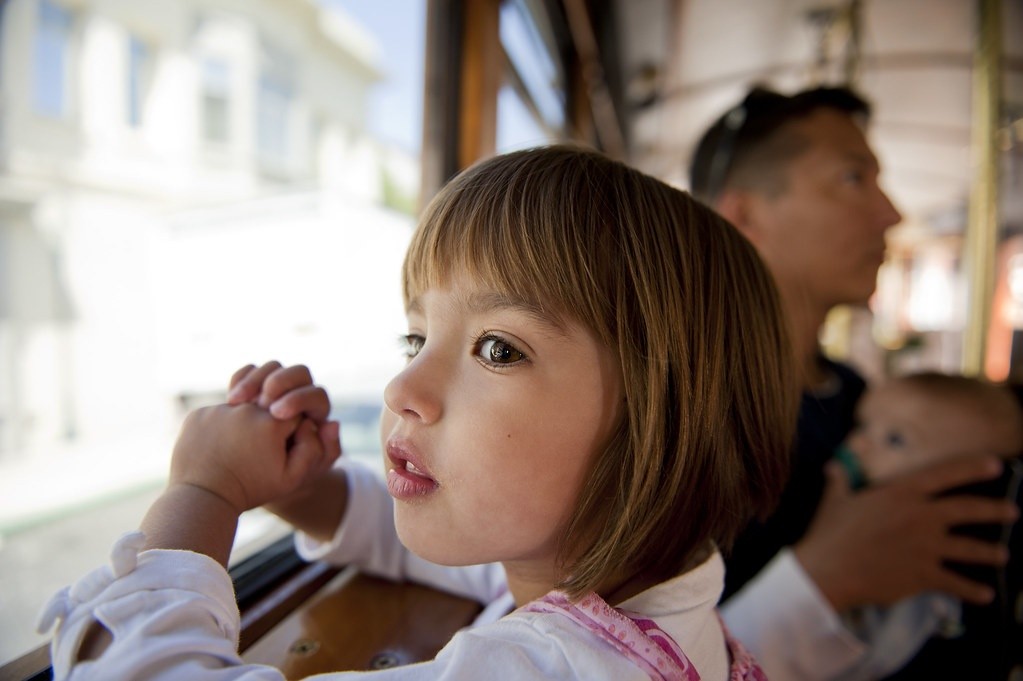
[839,366,1022,498]
[693,80,1023,680]
[34,143,790,680]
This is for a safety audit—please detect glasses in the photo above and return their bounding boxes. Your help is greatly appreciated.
[704,88,776,195]
